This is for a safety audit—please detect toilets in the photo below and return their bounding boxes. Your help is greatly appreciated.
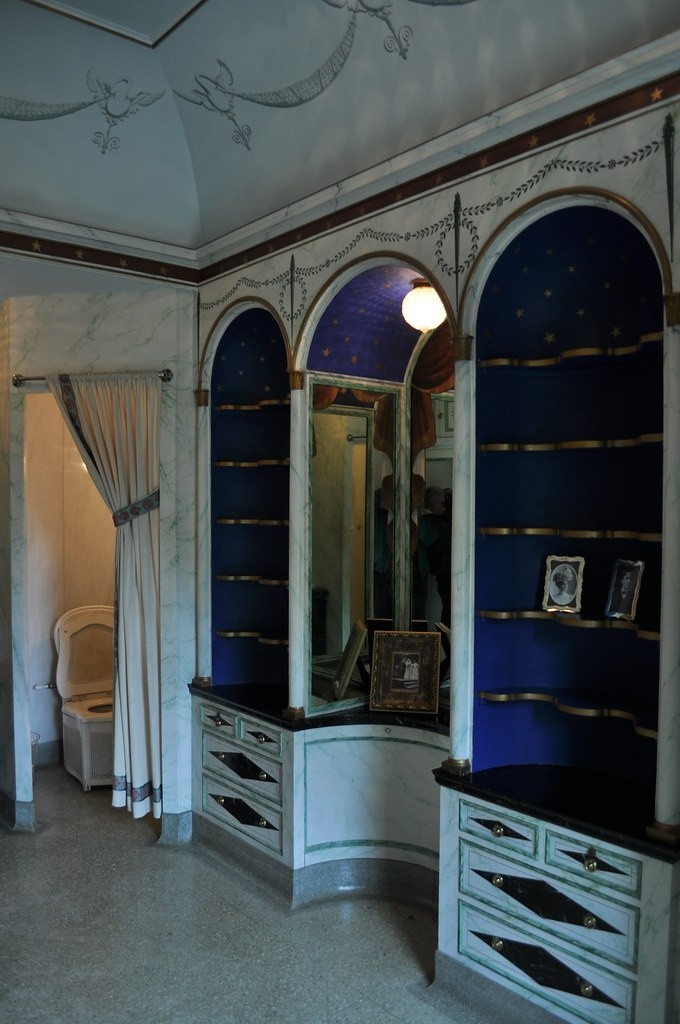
[50,601,118,794]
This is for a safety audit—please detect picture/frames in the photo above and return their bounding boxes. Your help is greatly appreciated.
[435,622,452,678]
[370,628,441,714]
[331,619,368,699]
[605,556,644,619]
[368,619,429,633]
[543,554,585,614]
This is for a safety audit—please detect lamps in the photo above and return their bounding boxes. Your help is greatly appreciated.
[402,278,448,333]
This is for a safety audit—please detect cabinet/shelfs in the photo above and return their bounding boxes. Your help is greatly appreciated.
[187,30,680,1024]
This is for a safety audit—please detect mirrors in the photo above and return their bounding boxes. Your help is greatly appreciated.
[306,317,454,723]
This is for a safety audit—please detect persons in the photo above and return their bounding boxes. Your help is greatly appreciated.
[552,572,573,603]
[397,656,419,679]
[608,568,633,616]
